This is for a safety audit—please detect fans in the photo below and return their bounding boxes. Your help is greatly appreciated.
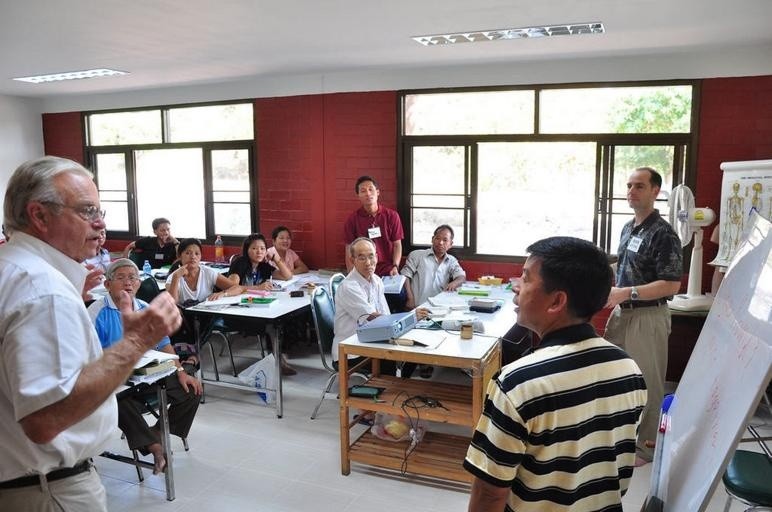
[669,184,715,313]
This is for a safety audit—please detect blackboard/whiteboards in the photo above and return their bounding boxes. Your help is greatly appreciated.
[646,206,772,512]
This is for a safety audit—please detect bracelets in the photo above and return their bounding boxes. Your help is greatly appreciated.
[175,367,184,372]
[223,290,227,296]
[276,258,281,265]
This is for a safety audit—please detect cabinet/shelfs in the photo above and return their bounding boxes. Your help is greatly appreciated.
[338,328,501,483]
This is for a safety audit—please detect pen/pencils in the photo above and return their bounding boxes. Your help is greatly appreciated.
[269,275,272,285]
[178,265,185,277]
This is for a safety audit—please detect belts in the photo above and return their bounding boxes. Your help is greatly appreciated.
[619,298,667,309]
[0,459,91,491]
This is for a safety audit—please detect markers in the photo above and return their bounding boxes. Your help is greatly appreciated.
[660,412,667,432]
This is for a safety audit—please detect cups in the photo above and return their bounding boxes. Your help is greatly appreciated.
[461,324,473,339]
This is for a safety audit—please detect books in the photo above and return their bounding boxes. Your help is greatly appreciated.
[126,349,180,386]
[205,296,241,306]
[240,297,279,307]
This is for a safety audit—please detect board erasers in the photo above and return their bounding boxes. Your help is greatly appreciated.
[647,496,663,512]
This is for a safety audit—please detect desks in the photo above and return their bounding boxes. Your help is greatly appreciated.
[666,309,708,382]
[97,374,174,502]
[88,261,231,294]
[411,281,533,360]
[185,270,347,418]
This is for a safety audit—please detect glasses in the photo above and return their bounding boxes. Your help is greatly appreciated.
[353,255,374,263]
[45,200,106,221]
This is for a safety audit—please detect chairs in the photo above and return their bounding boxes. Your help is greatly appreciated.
[120,395,189,481]
[212,319,264,377]
[199,339,219,379]
[330,273,347,303]
[311,286,370,420]
[723,449,772,512]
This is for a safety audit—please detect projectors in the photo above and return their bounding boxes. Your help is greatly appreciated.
[355,311,418,343]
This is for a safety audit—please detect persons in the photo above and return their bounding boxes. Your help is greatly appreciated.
[88,258,203,473]
[267,226,308,353]
[0,154,182,512]
[228,233,298,376]
[123,219,180,269]
[462,236,648,512]
[89,229,111,265]
[331,176,466,427]
[603,167,683,468]
[165,238,244,373]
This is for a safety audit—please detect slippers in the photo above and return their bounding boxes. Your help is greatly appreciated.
[353,414,374,427]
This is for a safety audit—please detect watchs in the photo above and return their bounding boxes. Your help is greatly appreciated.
[631,286,639,300]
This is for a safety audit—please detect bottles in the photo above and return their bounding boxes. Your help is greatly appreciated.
[142,260,152,280]
[213,235,225,262]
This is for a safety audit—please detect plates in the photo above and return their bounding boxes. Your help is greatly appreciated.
[478,278,504,285]
